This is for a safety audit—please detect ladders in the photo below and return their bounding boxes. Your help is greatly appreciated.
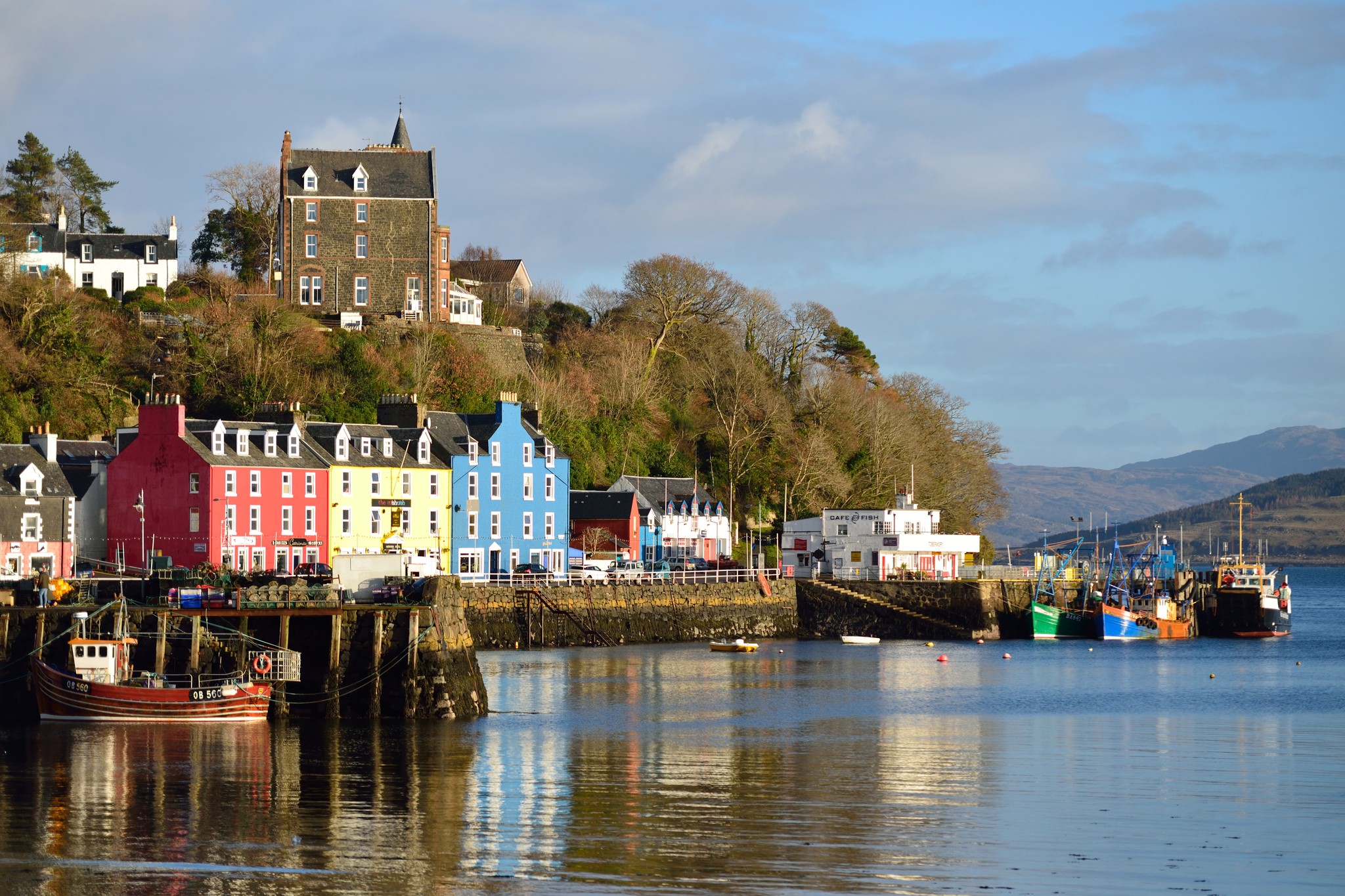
[79,577,91,602]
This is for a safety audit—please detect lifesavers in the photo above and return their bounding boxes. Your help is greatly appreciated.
[253,655,272,674]
[1136,617,1144,626]
[1147,619,1154,628]
[1152,622,1158,630]
[1142,617,1150,627]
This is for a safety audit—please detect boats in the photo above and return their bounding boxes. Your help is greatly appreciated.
[1088,519,1193,641]
[758,572,772,597]
[709,639,759,653]
[29,541,273,723]
[1019,528,1089,639]
[1205,493,1292,638]
[708,559,739,566]
[839,636,880,644]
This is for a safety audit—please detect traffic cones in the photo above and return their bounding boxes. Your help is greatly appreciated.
[729,573,735,582]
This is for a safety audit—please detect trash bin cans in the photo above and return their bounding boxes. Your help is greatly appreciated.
[152,556,173,569]
[735,565,748,582]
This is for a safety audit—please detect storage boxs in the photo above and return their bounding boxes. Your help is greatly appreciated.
[48,579,74,605]
[168,586,226,609]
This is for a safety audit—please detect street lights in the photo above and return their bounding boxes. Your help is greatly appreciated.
[1179,520,1184,564]
[745,501,762,553]
[1153,520,1162,554]
[1070,516,1084,579]
[133,488,146,604]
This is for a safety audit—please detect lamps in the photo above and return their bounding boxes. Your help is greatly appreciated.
[274,258,282,266]
[928,511,932,515]
[333,503,339,506]
[446,505,453,509]
[888,510,892,514]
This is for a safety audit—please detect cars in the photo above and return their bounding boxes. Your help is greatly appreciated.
[631,570,652,583]
[646,562,671,582]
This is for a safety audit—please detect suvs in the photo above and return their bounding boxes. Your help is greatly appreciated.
[678,557,709,573]
[604,559,644,585]
[71,561,95,579]
[644,556,696,574]
[567,564,609,586]
[513,563,555,584]
[294,562,333,575]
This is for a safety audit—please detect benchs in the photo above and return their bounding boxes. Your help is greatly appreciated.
[942,571,955,580]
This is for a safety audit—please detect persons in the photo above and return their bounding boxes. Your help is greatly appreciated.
[192,564,197,577]
[37,567,50,608]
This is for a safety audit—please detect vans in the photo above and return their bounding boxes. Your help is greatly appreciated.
[409,555,444,578]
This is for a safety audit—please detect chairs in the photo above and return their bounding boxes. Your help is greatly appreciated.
[895,566,932,580]
